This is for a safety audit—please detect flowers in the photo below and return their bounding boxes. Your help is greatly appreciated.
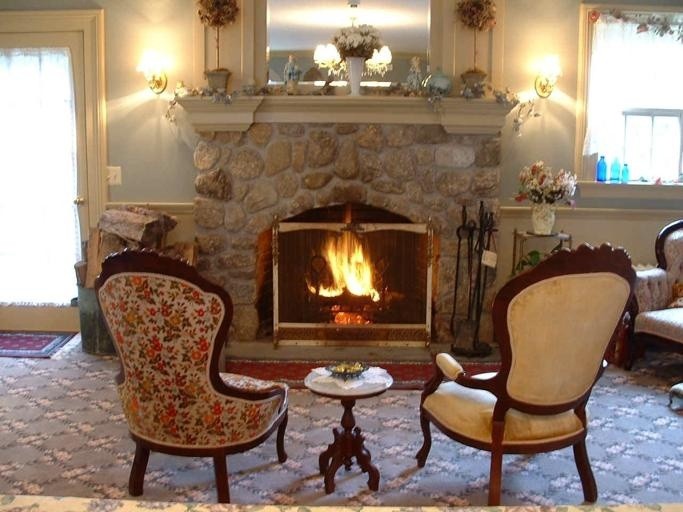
[514,160,577,205]
[331,27,377,59]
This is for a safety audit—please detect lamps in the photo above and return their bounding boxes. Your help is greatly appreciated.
[534,57,563,101]
[137,56,167,94]
[313,2,392,80]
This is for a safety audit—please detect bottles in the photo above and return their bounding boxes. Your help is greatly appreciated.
[610,157,621,183]
[596,155,607,182]
[621,162,630,182]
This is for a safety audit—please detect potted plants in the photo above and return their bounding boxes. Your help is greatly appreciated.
[198,0,240,92]
[456,0,499,94]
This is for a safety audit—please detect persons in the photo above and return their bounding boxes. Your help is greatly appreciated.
[405,55,423,90]
[283,54,303,95]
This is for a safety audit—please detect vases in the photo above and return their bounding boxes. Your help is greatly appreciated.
[344,59,368,97]
[530,203,555,235]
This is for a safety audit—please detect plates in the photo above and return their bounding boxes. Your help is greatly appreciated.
[325,360,370,377]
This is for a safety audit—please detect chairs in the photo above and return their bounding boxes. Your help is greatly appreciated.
[614,219,683,374]
[94,246,290,504]
[414,241,633,505]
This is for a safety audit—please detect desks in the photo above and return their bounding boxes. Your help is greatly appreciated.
[305,366,395,495]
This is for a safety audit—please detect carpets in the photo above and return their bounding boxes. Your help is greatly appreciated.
[2,327,78,359]
[222,359,503,391]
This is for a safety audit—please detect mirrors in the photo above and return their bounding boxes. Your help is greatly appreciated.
[253,0,442,94]
